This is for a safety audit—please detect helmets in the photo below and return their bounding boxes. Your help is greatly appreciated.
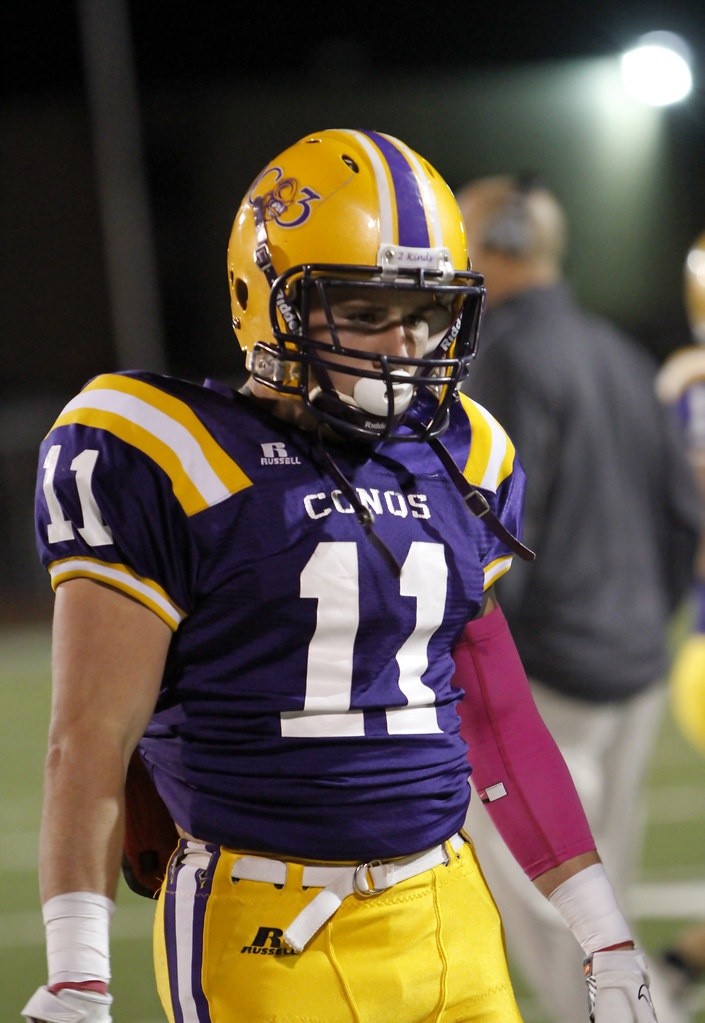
[226,129,471,429]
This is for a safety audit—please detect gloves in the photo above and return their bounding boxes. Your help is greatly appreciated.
[20,984,114,1022]
[582,948,656,1023]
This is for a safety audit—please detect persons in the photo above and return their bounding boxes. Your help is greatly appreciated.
[655,247,705,633]
[22,126,655,1022]
[455,170,679,1023]
